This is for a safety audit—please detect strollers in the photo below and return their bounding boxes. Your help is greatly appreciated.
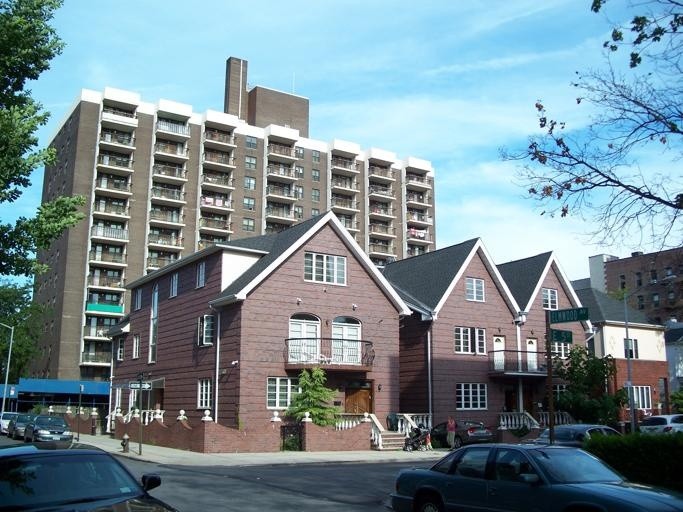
[403,426,430,452]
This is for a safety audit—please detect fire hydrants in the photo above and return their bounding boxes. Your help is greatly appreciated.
[120,433,129,454]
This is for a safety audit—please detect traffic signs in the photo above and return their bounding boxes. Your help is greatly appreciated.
[550,328,572,345]
[128,382,152,391]
[136,374,143,380]
[549,307,588,323]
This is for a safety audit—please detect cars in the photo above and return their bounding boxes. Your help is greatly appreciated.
[389,444,682,510]
[516,423,624,452]
[0,439,179,511]
[428,419,493,448]
[0,411,72,443]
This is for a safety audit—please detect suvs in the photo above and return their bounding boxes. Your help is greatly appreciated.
[640,413,683,435]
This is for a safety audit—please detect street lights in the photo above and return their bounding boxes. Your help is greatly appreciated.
[623,274,677,435]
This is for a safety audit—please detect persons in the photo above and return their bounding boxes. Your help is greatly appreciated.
[425,431,433,450]
[447,415,456,451]
